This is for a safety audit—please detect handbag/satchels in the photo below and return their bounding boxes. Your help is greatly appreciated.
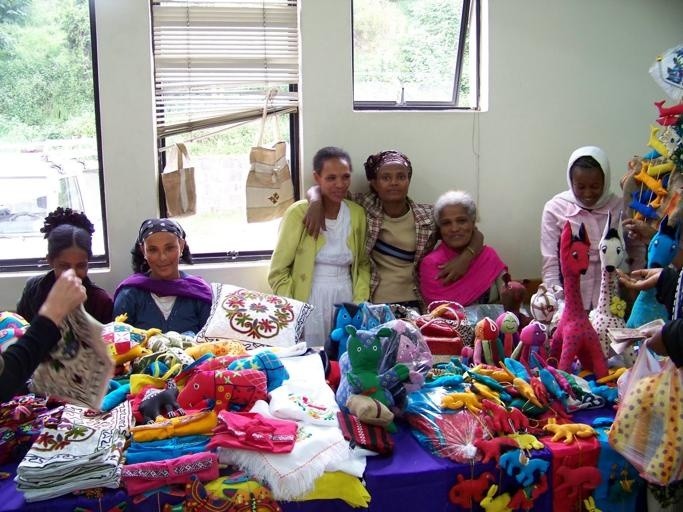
[244,87,297,224]
[158,142,198,220]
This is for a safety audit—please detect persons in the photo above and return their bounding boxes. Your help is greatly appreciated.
[414,189,508,309]
[612,97,683,511]
[539,145,626,312]
[299,149,486,313]
[13,205,110,331]
[266,146,372,354]
[106,214,211,333]
[0,265,86,404]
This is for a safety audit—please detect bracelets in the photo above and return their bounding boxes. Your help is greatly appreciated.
[465,246,477,258]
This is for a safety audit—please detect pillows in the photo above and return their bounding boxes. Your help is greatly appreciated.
[195,282,314,349]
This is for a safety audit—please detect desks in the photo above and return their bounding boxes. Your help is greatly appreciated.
[0,404,649,512]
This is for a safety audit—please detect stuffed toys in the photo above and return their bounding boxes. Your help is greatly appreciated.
[313,269,626,512]
[314,270,626,512]
[549,218,608,382]
[87,312,276,432]
[587,208,627,362]
